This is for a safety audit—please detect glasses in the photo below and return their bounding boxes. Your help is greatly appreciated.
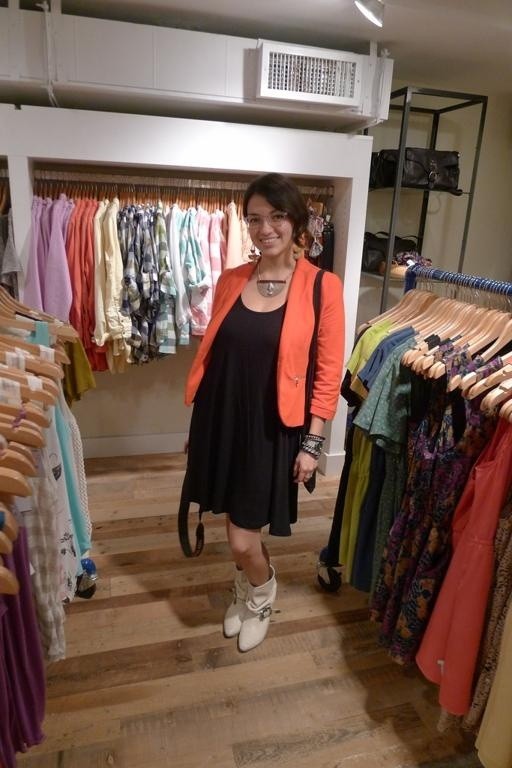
[246,211,287,226]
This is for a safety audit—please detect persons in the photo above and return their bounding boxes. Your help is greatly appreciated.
[177,172,347,655]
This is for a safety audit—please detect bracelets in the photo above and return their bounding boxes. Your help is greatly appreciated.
[299,433,326,458]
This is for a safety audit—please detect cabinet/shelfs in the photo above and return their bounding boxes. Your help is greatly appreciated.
[354,82,488,317]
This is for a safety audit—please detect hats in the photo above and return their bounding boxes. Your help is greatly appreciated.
[301,414,318,494]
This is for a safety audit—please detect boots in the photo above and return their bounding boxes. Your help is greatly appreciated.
[237,563,278,654]
[222,565,250,640]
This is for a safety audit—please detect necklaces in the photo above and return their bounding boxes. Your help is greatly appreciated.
[257,261,295,296]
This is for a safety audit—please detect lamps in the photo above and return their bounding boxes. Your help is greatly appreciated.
[355,0,388,28]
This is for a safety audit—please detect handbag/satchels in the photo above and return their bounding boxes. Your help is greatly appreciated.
[361,230,421,276]
[400,146,464,196]
[367,149,398,189]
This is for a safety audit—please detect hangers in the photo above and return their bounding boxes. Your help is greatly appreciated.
[1,279,81,610]
[1,169,14,218]
[361,262,512,421]
